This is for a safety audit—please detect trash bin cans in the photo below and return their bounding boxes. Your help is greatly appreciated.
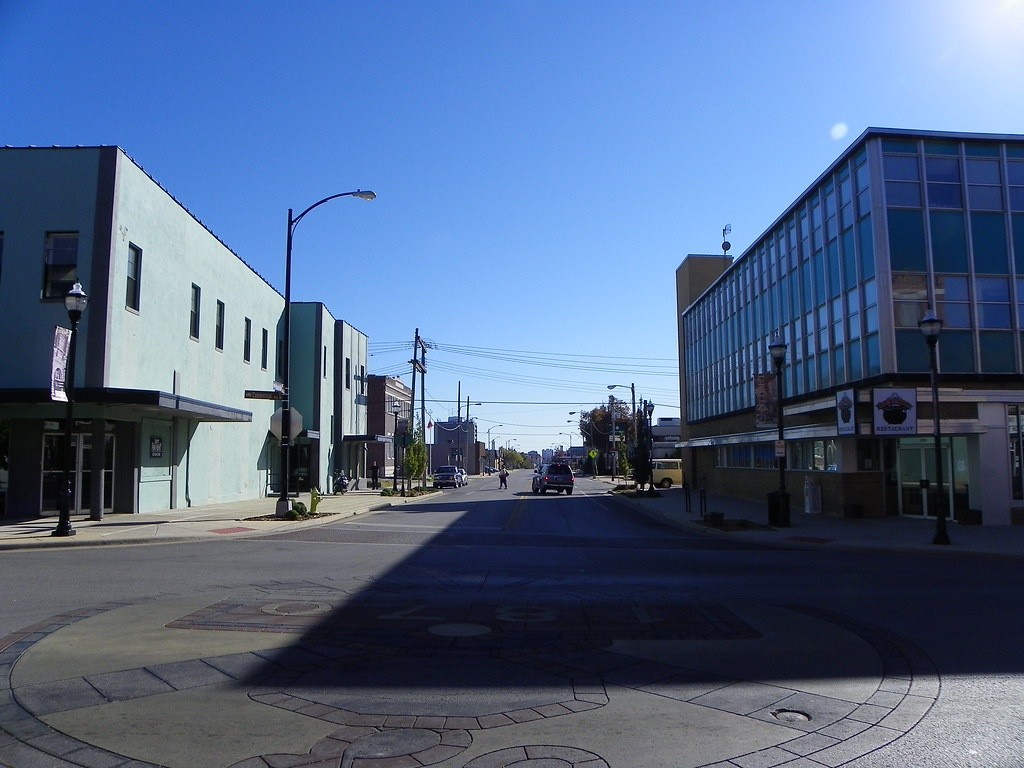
[767,492,791,528]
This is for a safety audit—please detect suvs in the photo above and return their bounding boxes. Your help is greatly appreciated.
[433,465,463,489]
[532,461,575,495]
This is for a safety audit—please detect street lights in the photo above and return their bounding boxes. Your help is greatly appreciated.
[487,425,503,476]
[550,411,596,479]
[276,188,378,517]
[457,402,482,469]
[49,276,90,538]
[767,330,793,527]
[391,401,401,493]
[506,439,517,456]
[606,382,638,449]
[918,302,952,546]
[646,399,659,497]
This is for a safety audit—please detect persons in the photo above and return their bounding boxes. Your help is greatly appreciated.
[498,465,508,489]
[370,460,379,490]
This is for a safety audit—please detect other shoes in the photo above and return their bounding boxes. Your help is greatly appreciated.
[498,488,502,489]
[504,488,508,489]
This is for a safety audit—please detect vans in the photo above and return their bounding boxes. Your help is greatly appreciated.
[645,459,683,489]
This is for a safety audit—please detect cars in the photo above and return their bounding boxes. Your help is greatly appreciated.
[457,468,468,487]
[485,466,500,473]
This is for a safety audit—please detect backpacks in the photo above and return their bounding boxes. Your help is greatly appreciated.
[506,470,509,477]
